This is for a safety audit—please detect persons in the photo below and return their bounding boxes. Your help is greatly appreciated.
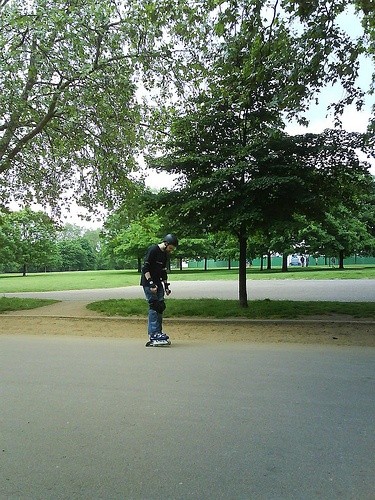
[301,255,309,267]
[140,234,178,342]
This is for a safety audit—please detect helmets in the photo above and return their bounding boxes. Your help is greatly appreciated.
[163,234,178,247]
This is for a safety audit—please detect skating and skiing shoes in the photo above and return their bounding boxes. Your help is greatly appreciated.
[145,332,171,347]
